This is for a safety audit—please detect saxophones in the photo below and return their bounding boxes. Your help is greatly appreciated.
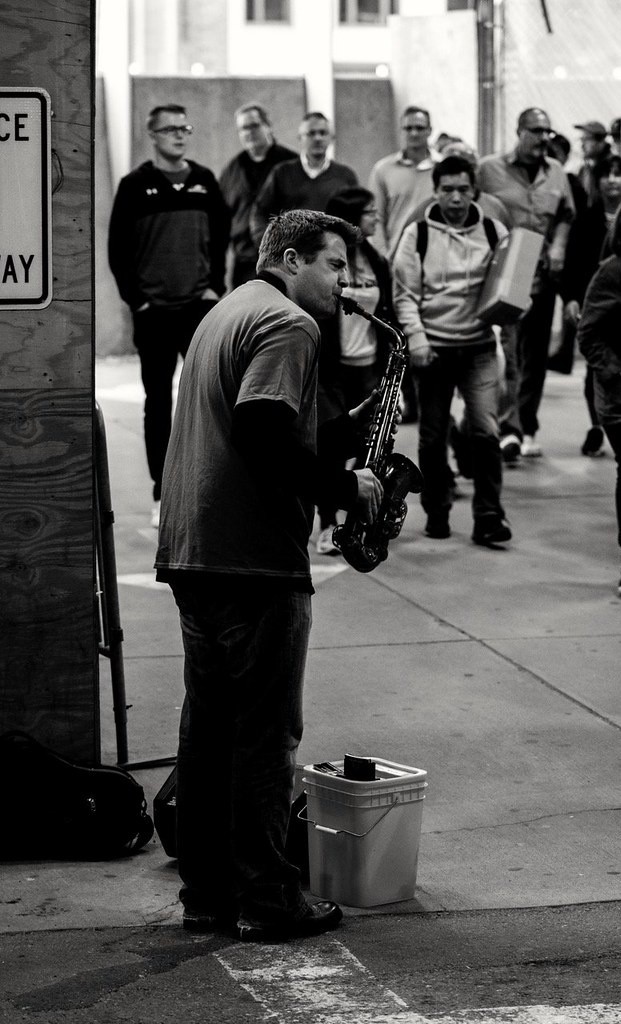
[331,292,424,577]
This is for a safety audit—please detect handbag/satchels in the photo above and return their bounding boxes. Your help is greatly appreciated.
[0,730,154,862]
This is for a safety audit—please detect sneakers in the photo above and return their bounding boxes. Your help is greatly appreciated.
[317,524,342,554]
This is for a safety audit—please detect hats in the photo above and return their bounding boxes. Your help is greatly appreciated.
[574,121,608,142]
[609,119,621,135]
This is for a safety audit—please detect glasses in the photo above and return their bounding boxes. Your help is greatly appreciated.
[362,207,376,216]
[523,124,557,140]
[154,124,193,134]
[402,125,426,132]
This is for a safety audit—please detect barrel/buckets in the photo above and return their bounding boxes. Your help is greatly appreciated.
[297,754,428,908]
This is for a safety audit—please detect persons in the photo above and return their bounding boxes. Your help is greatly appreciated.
[367,105,459,426]
[248,111,361,252]
[476,106,621,608]
[216,100,300,295]
[293,186,400,556]
[390,133,515,546]
[107,102,234,530]
[152,208,385,944]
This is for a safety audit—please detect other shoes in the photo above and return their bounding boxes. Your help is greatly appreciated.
[471,514,512,544]
[238,901,342,941]
[426,510,450,538]
[182,915,240,931]
[521,435,541,457]
[582,427,604,456]
[499,434,520,463]
[546,352,575,374]
[151,501,160,527]
[402,399,418,424]
